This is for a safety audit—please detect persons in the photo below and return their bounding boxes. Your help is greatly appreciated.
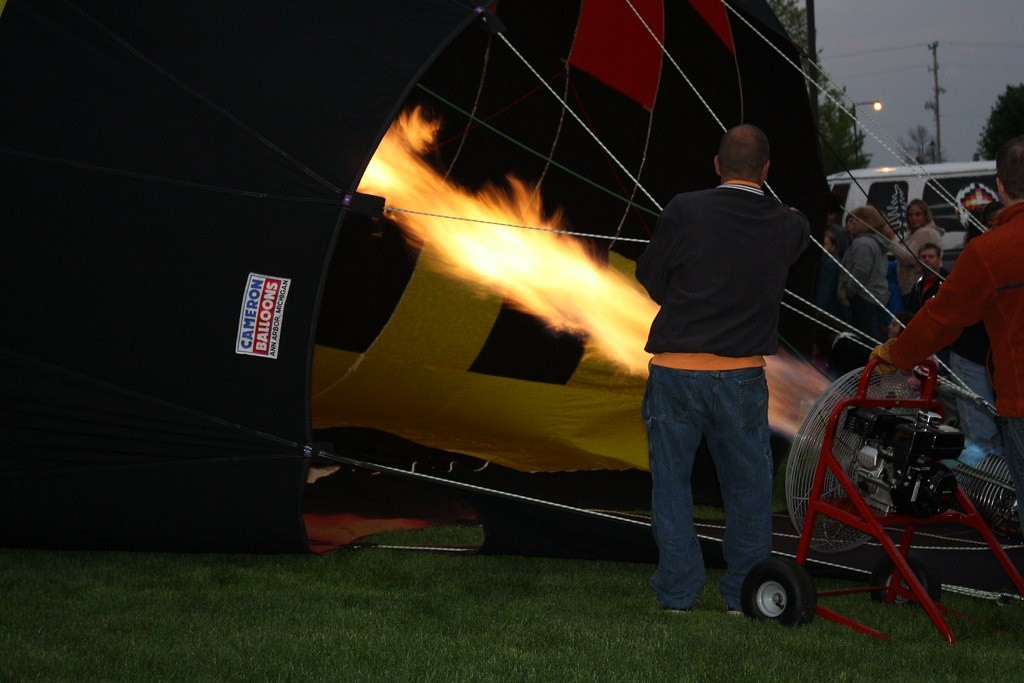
[635,125,810,619]
[821,137,1024,540]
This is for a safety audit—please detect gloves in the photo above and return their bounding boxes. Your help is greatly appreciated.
[868,337,898,375]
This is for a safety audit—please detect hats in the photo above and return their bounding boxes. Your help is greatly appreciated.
[828,223,850,257]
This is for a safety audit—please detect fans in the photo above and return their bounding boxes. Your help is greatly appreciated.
[951,445,1017,525]
[784,366,928,551]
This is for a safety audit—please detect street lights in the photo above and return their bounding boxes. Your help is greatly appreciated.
[853,101,883,157]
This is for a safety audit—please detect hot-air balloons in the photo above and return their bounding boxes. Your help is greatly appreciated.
[1,1,1024,600]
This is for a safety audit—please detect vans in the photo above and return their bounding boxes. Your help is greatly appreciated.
[826,160,1001,270]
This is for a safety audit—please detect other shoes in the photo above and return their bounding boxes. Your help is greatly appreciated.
[660,599,701,612]
[724,602,744,616]
